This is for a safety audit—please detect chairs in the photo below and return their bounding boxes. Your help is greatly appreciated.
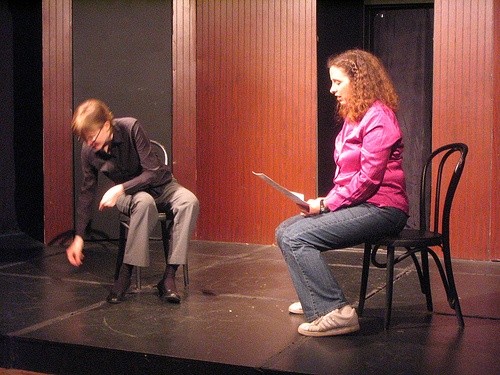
[358,142,469,331]
[114,139,190,291]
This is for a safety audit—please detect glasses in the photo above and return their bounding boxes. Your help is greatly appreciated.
[77,122,106,144]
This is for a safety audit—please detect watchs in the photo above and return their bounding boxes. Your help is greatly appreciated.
[319,199,328,214]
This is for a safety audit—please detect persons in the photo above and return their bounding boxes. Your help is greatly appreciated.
[66,99,200,303]
[275,48,409,337]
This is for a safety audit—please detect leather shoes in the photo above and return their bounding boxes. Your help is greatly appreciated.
[157,279,181,304]
[107,281,131,304]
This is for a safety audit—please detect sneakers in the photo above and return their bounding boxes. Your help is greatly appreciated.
[297,307,360,337]
[288,302,305,315]
